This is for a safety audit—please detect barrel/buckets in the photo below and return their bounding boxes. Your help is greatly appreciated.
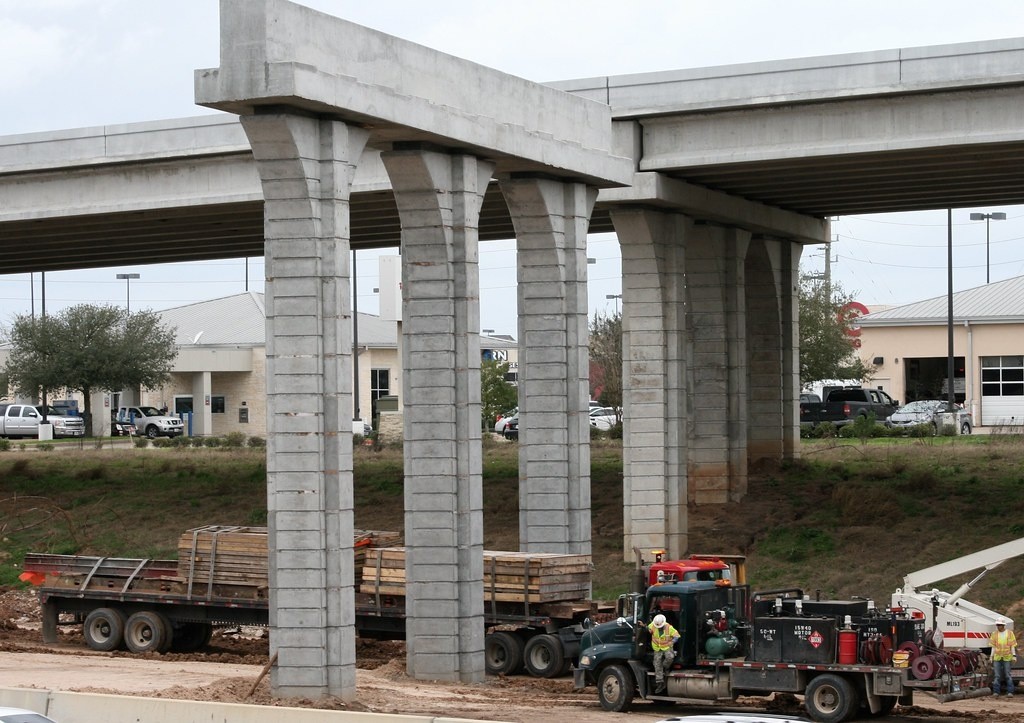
[893,649,910,668]
[838,628,857,664]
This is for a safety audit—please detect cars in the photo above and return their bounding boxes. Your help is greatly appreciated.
[505,411,518,441]
[888,399,974,439]
[111,417,139,439]
[494,407,517,436]
[590,407,622,432]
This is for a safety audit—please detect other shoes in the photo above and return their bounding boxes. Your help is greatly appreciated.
[992,692,999,697]
[662,667,667,676]
[1007,692,1013,698]
[654,682,666,693]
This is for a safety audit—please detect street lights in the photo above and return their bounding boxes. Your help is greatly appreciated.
[117,274,140,315]
[606,295,623,318]
[972,212,1007,286]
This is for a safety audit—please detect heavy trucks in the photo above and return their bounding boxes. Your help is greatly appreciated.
[19,518,737,678]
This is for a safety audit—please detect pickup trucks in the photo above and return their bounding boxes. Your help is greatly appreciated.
[0,402,86,439]
[799,387,901,436]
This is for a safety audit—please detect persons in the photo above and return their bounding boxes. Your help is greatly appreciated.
[988,617,1017,697]
[638,614,681,693]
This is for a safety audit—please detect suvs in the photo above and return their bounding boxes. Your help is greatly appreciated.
[117,406,184,438]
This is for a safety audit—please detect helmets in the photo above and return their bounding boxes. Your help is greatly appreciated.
[995,618,1007,625]
[652,613,667,628]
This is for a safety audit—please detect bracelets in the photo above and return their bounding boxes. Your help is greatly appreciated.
[1013,655,1017,657]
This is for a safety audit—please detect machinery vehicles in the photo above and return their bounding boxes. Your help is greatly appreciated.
[576,582,996,722]
[888,537,1024,687]
